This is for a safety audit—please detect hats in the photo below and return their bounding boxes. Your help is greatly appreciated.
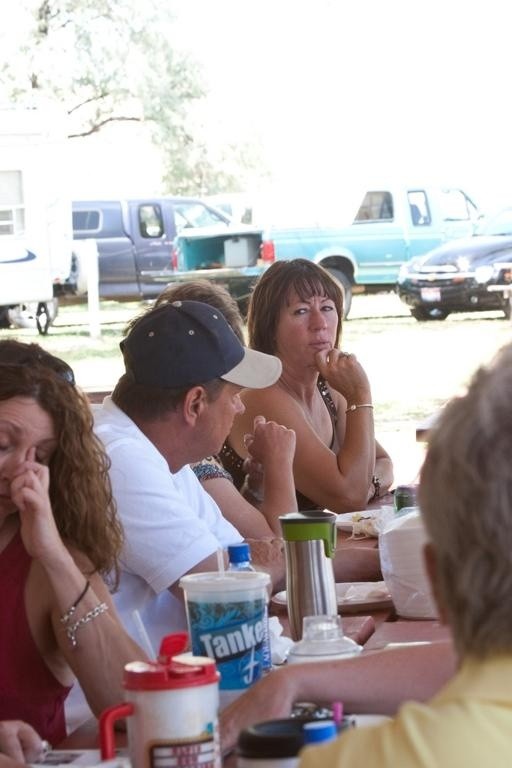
[118,300,284,391]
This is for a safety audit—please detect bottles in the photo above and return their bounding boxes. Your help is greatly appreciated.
[300,717,344,747]
[393,484,416,512]
[287,612,361,665]
[222,542,273,677]
[238,718,335,768]
[277,512,341,642]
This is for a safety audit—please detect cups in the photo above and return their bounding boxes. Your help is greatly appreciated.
[98,630,221,767]
[180,572,273,712]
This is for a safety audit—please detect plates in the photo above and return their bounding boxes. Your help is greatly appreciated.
[272,581,399,615]
[333,510,392,530]
